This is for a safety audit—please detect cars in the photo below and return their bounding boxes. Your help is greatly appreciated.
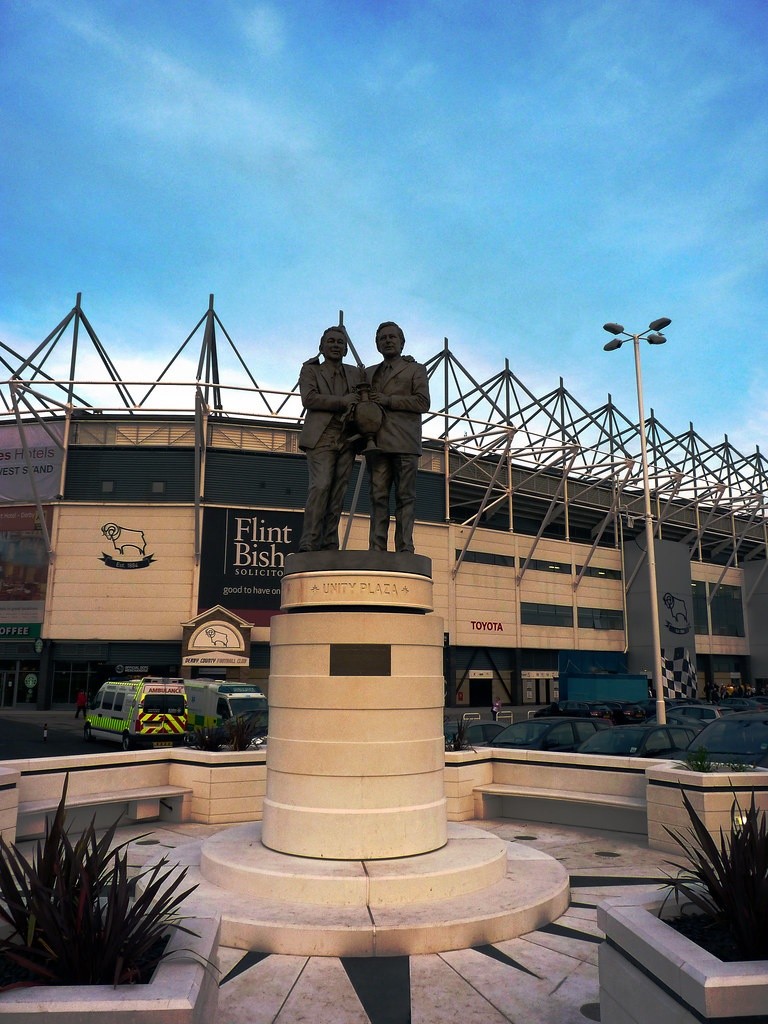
[444,696,768,773]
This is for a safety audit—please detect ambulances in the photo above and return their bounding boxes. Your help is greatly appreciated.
[82,674,191,752]
[182,678,264,743]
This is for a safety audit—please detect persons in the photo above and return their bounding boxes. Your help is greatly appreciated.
[703,681,768,703]
[74,690,87,720]
[491,696,502,721]
[299,321,431,554]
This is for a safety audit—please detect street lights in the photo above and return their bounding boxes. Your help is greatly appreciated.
[601,316,672,728]
